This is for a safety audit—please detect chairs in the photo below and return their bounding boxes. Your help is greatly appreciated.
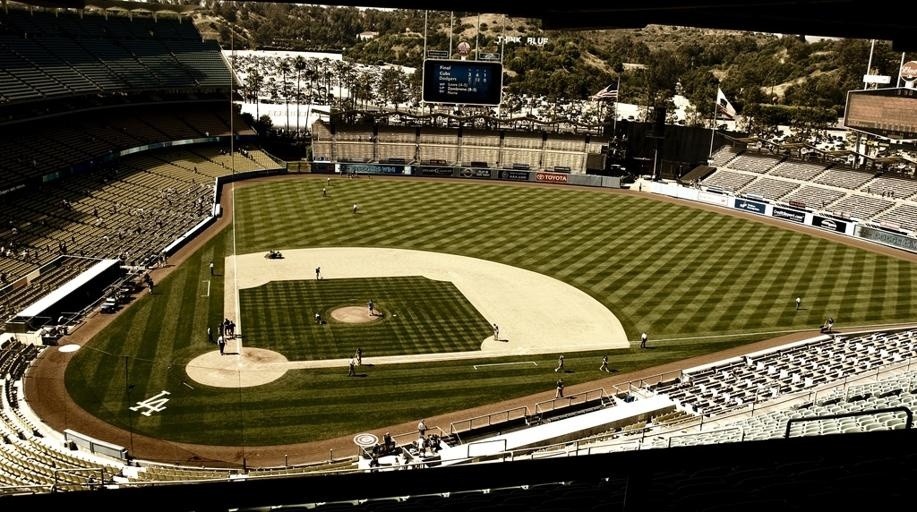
[530,332,917,453]
[119,464,231,488]
[248,460,360,478]
[0,409,119,494]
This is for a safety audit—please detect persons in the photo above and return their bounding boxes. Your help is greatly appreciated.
[218,147,253,160]
[828,317,834,333]
[369,420,436,472]
[322,169,371,214]
[86,474,96,490]
[60,166,213,262]
[316,267,320,280]
[493,324,499,340]
[368,299,374,316]
[795,296,801,310]
[555,378,564,398]
[157,252,168,268]
[1,216,67,316]
[314,313,322,324]
[555,356,564,372]
[144,272,154,294]
[207,318,236,355]
[39,325,46,338]
[266,248,281,258]
[347,348,361,376]
[640,332,647,347]
[599,353,610,372]
[209,260,214,274]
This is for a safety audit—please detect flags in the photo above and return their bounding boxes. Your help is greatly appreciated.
[587,82,617,102]
[716,87,737,120]
